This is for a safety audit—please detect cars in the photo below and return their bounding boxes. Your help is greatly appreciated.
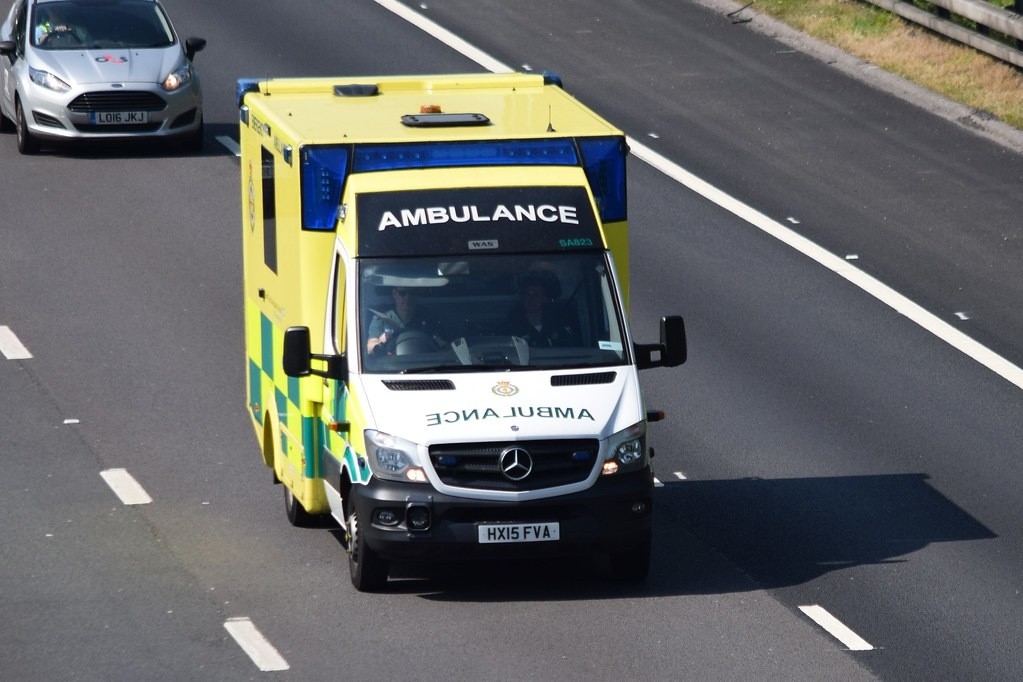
[1,0,209,156]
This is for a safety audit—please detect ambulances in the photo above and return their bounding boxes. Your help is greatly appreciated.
[237,71,690,593]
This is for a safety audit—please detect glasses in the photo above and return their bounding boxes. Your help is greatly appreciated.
[395,289,419,297]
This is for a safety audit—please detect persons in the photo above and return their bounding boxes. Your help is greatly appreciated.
[499,276,574,350]
[33,3,95,48]
[366,281,448,358]
